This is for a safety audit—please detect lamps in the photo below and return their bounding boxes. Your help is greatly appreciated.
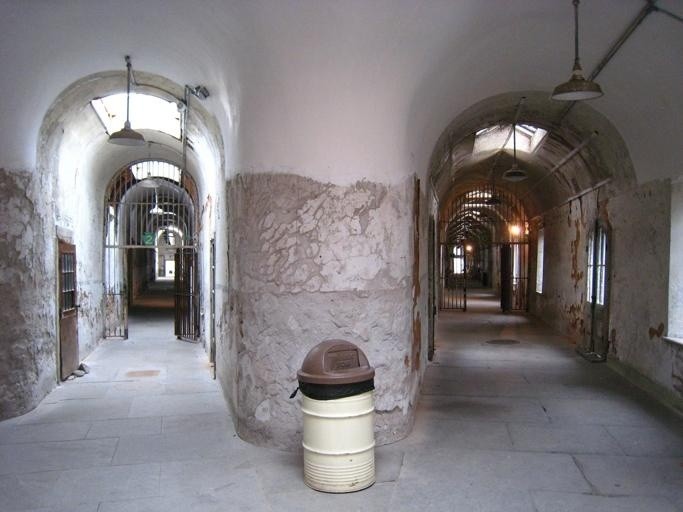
[139,140,173,244]
[502,119,527,182]
[550,0,604,100]
[457,166,501,248]
[105,55,146,147]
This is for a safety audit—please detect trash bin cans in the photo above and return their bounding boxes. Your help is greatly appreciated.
[296,339,375,494]
